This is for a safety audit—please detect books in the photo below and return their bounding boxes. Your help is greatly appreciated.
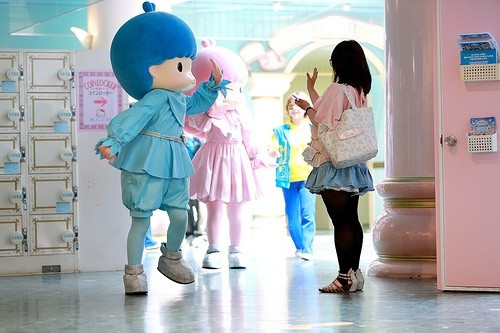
[458,32,500,65]
[470,117,496,135]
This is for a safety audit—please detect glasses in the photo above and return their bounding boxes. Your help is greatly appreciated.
[329,59,332,66]
[288,105,294,111]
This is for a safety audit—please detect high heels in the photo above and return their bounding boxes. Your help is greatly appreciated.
[319,272,358,294]
[353,269,363,290]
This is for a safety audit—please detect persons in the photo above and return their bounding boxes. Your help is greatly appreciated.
[268,92,317,260]
[294,40,376,293]
[181,40,260,269]
[93,1,230,293]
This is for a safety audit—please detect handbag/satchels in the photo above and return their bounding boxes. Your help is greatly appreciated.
[318,84,378,170]
[302,147,322,168]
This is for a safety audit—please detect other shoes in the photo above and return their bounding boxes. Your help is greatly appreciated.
[301,253,309,261]
[295,250,301,257]
[187,234,208,248]
[147,243,159,250]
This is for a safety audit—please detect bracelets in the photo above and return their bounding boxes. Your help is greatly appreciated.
[306,106,314,114]
